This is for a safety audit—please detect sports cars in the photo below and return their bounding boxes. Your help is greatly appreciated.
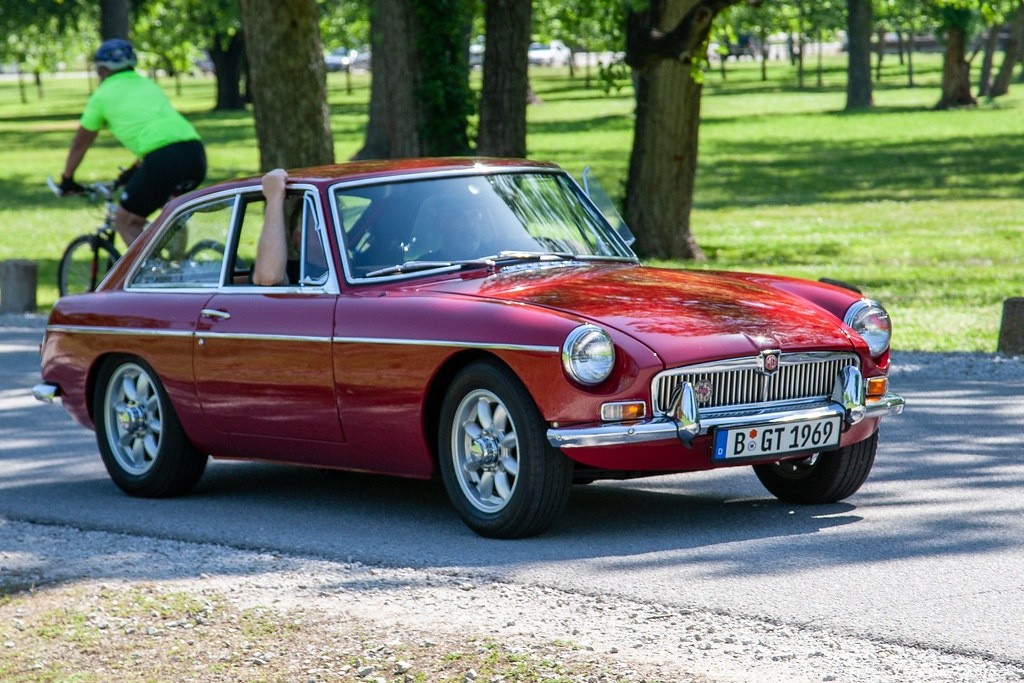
[28,153,908,543]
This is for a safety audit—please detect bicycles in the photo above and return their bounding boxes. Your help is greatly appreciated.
[45,172,247,299]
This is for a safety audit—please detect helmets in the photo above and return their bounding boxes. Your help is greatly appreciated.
[94,38,138,71]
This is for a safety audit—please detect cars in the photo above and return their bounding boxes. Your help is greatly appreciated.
[325,44,373,72]
[722,33,768,61]
[528,38,574,66]
[468,35,485,69]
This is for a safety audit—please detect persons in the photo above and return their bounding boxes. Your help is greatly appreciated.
[250,169,363,286]
[412,190,589,262]
[60,39,207,260]
